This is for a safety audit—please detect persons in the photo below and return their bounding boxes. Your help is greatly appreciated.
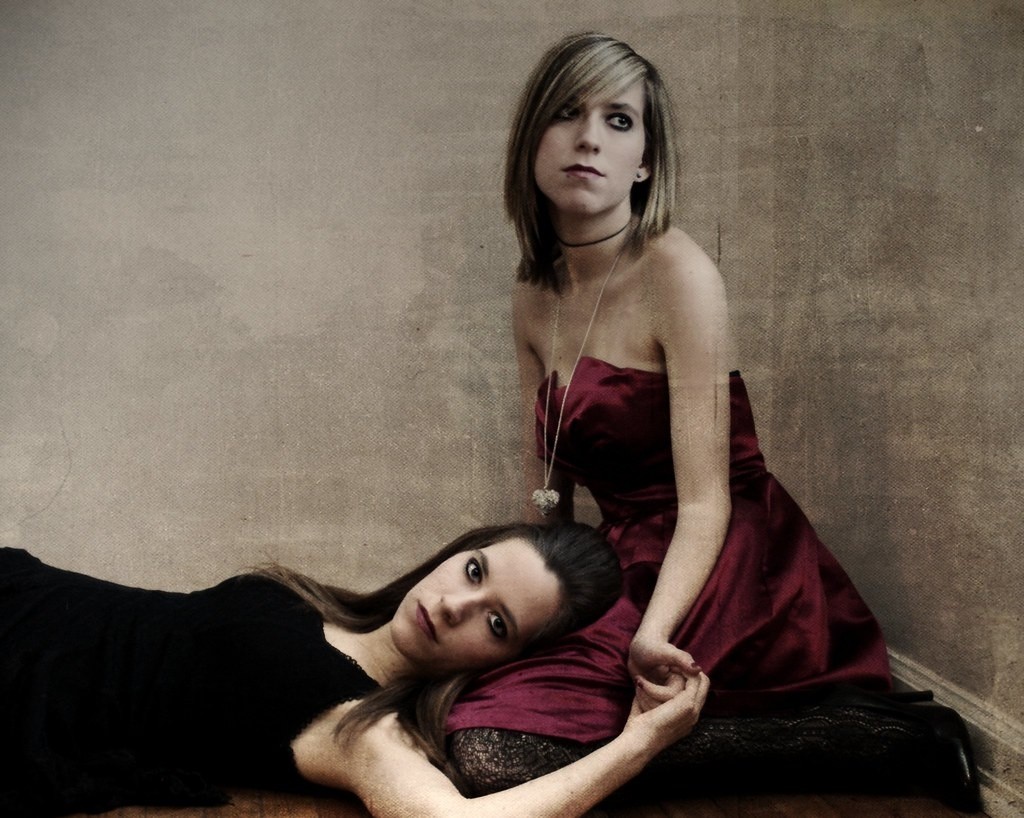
[436,34,984,818]
[0,521,710,818]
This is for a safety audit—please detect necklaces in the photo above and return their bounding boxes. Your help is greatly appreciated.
[532,252,623,516]
[557,225,629,247]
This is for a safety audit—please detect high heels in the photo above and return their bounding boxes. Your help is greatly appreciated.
[817,689,980,813]
